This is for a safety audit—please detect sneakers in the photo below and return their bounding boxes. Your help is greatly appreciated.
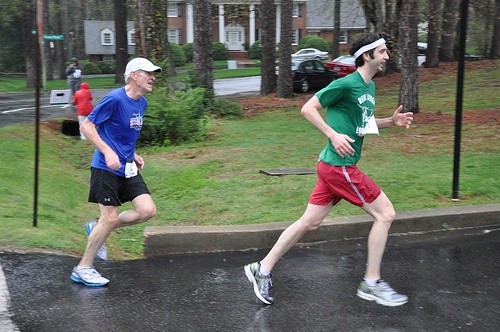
[244,261,274,305]
[69,266,110,287]
[86,221,109,260]
[356,278,408,307]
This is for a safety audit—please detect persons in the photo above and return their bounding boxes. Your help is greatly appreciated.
[72,82,94,140]
[243,33,414,307]
[64,57,82,110]
[69,57,163,287]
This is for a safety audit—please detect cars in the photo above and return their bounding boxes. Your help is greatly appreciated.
[290,48,328,61]
[399,42,428,66]
[275,58,340,93]
[326,55,382,80]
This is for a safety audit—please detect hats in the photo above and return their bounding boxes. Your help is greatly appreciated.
[71,57,79,62]
[124,56,162,84]
